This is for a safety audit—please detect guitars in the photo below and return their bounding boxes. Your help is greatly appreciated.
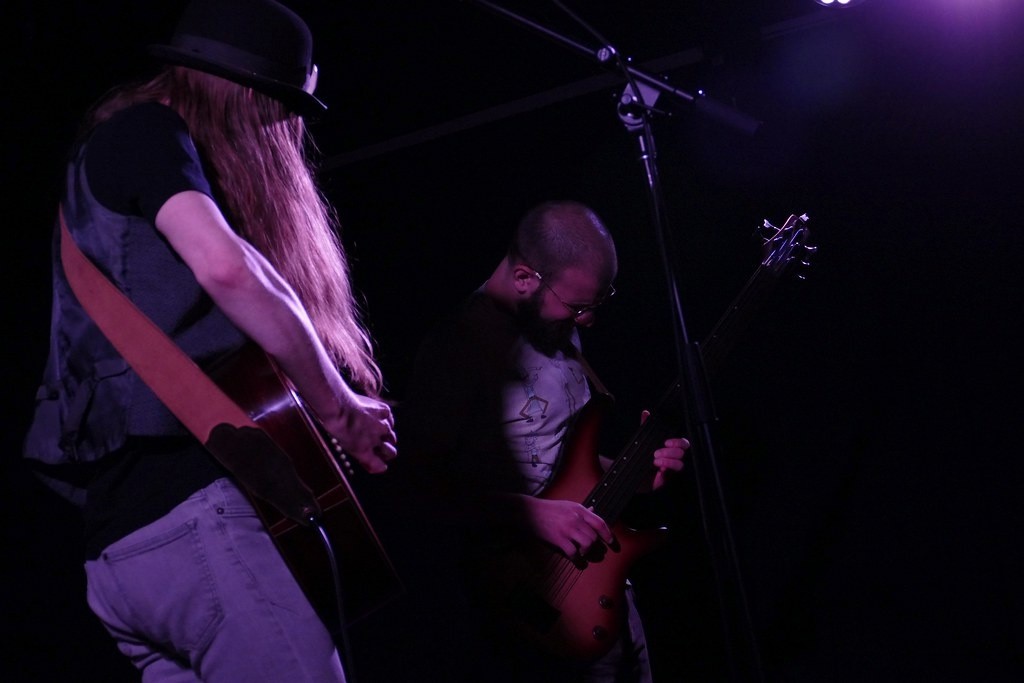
[479,208,823,683]
[239,338,402,621]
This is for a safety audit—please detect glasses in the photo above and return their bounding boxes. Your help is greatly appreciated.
[535,271,616,314]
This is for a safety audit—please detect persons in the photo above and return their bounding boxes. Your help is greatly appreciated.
[25,3,399,683]
[409,201,692,683]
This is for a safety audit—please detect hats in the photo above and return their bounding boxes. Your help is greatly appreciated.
[154,0,329,116]
